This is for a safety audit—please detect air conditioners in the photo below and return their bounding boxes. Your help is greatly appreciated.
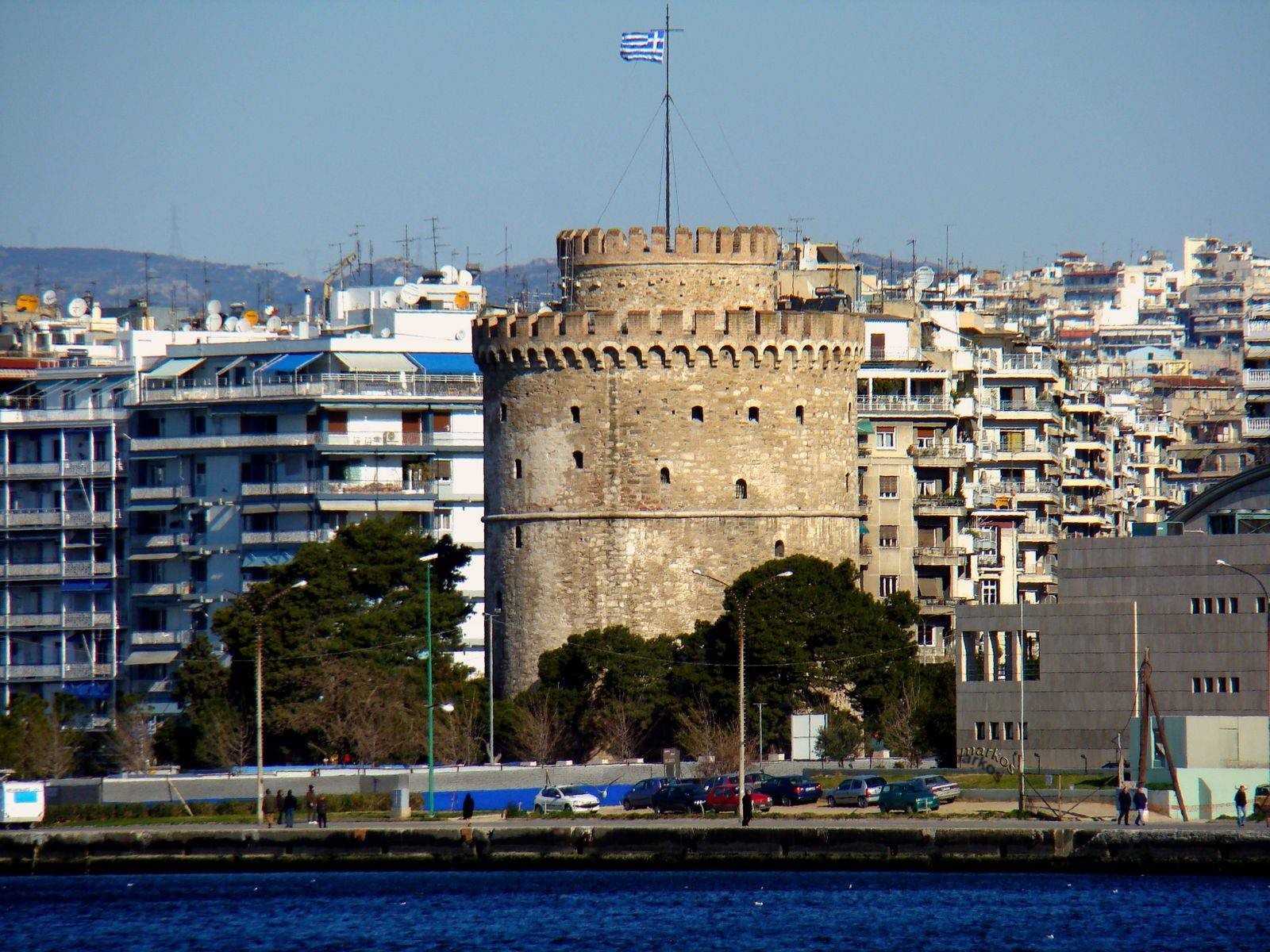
[385,431,399,442]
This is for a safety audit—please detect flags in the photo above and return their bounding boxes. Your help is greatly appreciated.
[620,28,665,64]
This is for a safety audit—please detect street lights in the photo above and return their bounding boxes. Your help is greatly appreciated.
[221,578,311,827]
[753,703,770,773]
[417,551,455,820]
[1034,751,1040,774]
[1214,558,1270,770]
[1079,753,1089,775]
[692,567,794,822]
[1012,750,1018,770]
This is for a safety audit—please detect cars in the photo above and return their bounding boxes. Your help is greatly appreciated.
[825,774,889,807]
[622,776,682,811]
[906,774,961,803]
[878,781,941,814]
[533,783,601,815]
[1253,785,1270,815]
[651,782,710,812]
[1102,763,1130,781]
[760,774,823,806]
[704,769,775,791]
[705,782,774,813]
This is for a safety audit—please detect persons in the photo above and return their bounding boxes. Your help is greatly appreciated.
[1234,785,1247,827]
[1118,786,1131,826]
[463,793,474,820]
[262,788,298,828]
[1133,787,1147,826]
[306,784,328,828]
[330,749,352,765]
[742,788,753,827]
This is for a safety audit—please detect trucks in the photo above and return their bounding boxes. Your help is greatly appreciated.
[0,768,45,831]
